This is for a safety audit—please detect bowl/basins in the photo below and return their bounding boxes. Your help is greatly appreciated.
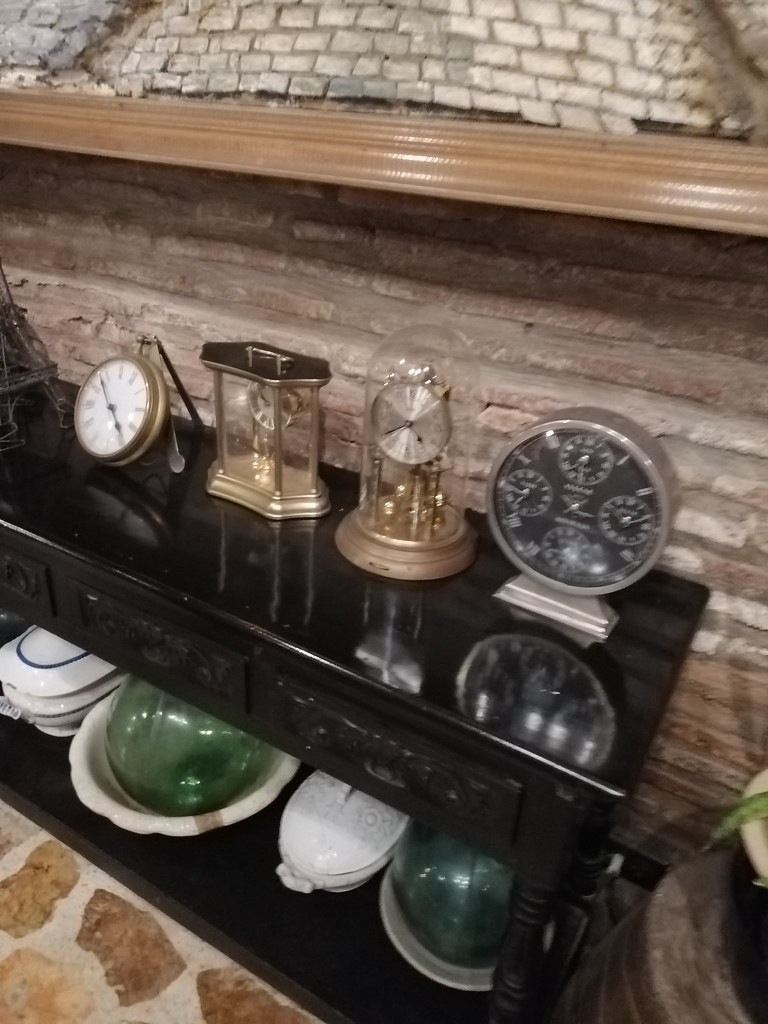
[65,690,300,838]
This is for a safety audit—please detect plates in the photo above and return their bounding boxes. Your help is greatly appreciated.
[377,862,555,994]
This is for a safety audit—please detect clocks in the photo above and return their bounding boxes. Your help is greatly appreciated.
[197,339,334,521]
[454,607,630,771]
[485,404,681,639]
[73,335,187,473]
[335,323,489,581]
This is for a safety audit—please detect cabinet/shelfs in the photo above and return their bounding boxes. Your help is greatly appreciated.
[1,375,711,1024]
